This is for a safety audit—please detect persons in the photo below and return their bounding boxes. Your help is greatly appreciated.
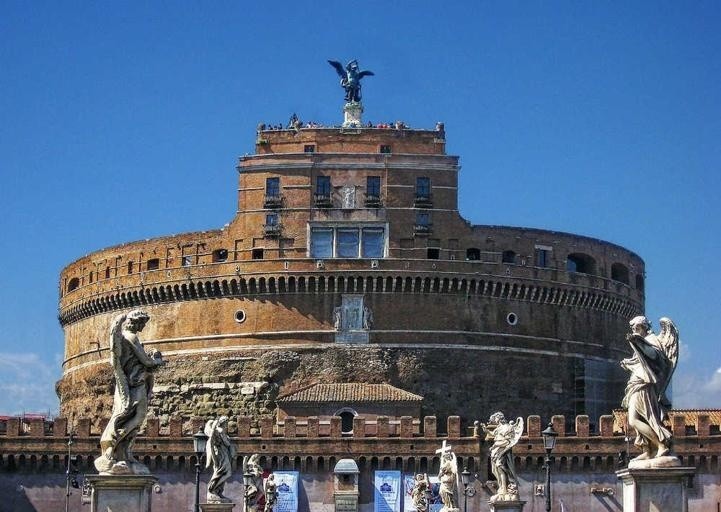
[438,452,458,507]
[243,454,279,512]
[204,416,233,500]
[620,316,679,460]
[100,309,169,461]
[480,411,524,495]
[266,110,411,128]
[412,473,432,512]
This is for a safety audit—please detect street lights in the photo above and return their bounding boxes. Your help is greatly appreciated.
[191,426,210,512]
[542,422,560,511]
[462,466,471,512]
